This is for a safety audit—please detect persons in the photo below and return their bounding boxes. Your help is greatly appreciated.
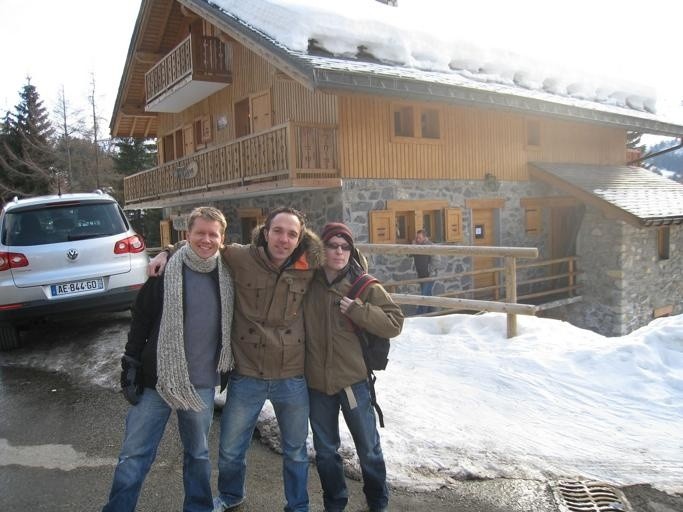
[146,207,326,512]
[103,206,235,512]
[412,230,441,314]
[301,223,404,512]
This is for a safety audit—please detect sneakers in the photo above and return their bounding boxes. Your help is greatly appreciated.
[211,496,246,511]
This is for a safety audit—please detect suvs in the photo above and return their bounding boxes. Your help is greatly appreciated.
[0,189,150,350]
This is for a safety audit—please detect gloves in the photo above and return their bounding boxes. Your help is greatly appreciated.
[121,355,144,406]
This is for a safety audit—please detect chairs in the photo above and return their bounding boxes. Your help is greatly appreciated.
[17,214,42,240]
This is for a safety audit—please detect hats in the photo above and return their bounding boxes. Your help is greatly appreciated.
[320,222,354,244]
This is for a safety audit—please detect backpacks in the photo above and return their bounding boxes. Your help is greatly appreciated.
[347,274,390,370]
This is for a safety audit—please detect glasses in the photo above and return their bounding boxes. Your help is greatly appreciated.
[324,242,352,250]
